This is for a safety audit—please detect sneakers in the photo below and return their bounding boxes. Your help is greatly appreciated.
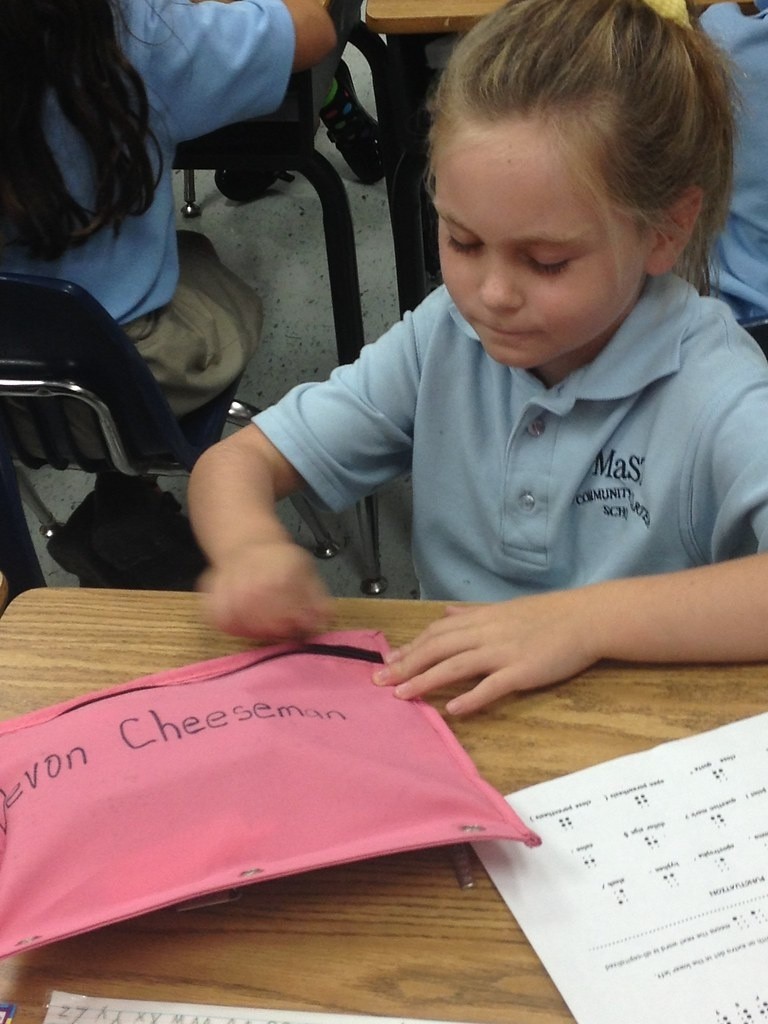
[213,161,295,203]
[319,58,385,184]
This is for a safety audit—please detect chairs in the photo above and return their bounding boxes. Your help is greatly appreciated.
[0,268,335,594]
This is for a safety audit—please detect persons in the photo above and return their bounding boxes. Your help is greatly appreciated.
[185,0,768,714]
[644,0,768,319]
[212,54,386,203]
[0,0,340,467]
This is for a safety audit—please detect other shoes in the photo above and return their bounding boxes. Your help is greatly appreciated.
[44,491,203,592]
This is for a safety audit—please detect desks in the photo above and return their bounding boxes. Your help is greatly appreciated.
[0,586,768,1024]
[167,1,389,368]
[364,0,689,322]
